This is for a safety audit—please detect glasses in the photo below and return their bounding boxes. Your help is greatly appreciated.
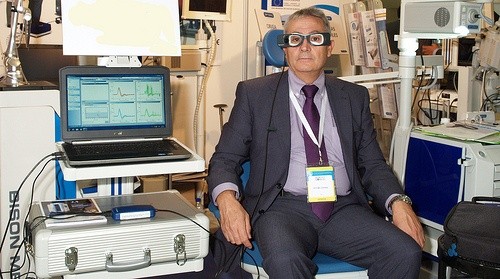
[277,32,331,48]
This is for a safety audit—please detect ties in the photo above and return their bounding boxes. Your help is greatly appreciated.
[301,85,335,222]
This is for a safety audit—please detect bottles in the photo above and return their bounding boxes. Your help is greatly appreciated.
[194,198,203,212]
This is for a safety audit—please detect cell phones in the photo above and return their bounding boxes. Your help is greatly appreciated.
[113,204,154,221]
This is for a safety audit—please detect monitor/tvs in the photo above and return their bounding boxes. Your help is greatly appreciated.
[181,0,233,22]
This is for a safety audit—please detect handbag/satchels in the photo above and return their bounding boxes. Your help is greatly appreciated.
[437,196,500,279]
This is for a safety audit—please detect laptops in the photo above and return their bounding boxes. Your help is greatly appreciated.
[59,66,191,166]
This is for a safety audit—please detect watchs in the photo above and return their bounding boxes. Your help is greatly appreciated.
[389,194,412,209]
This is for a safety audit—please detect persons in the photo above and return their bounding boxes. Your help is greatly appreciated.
[207,8,425,279]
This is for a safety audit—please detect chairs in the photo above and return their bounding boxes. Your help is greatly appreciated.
[208,29,371,279]
